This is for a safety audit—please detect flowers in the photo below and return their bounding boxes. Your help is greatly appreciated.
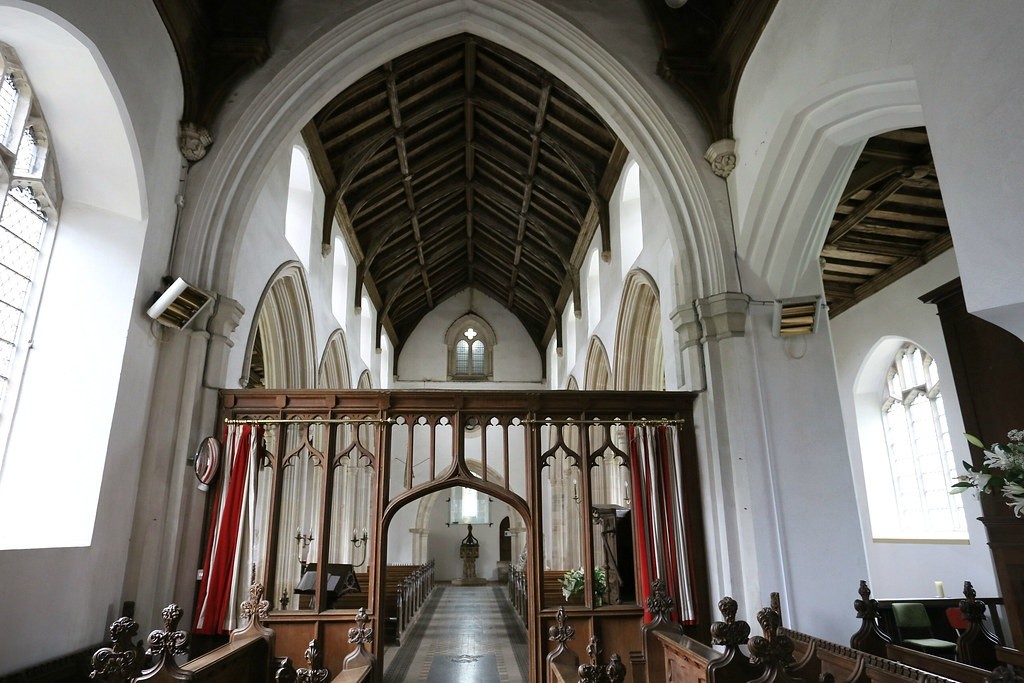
[942,420,1023,521]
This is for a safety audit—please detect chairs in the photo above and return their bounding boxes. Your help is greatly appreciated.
[946,604,973,644]
[890,600,955,660]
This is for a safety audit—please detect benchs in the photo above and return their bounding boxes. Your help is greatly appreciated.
[299,558,438,647]
[503,563,579,630]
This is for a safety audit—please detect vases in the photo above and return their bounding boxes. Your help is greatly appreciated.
[556,564,611,608]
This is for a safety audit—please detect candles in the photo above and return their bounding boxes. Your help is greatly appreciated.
[934,580,946,600]
